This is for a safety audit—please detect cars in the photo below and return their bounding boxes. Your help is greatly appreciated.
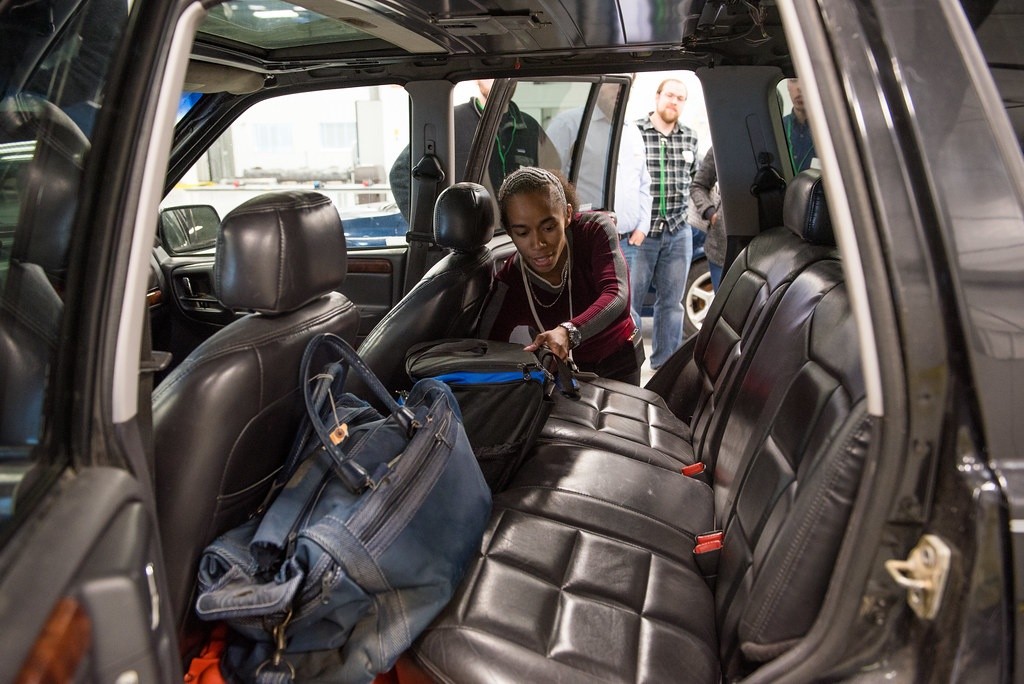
[0,0,1024,682]
[338,200,714,338]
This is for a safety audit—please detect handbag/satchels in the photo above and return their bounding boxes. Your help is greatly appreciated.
[396,339,581,496]
[197,332,493,684]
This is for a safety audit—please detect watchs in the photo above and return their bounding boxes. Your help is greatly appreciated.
[559,321,582,350]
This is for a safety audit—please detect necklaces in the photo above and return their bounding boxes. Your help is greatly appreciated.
[476,98,517,176]
[522,257,569,308]
[787,117,814,176]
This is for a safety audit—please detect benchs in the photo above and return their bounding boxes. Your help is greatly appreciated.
[412,169,875,684]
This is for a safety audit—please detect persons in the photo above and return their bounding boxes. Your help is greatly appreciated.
[689,146,727,297]
[546,71,654,277]
[469,165,643,388]
[780,76,818,176]
[632,77,699,375]
[388,79,563,224]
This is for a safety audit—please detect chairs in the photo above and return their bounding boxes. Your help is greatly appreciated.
[357,181,498,386]
[152,192,361,649]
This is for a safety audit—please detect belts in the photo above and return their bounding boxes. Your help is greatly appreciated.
[618,232,632,241]
